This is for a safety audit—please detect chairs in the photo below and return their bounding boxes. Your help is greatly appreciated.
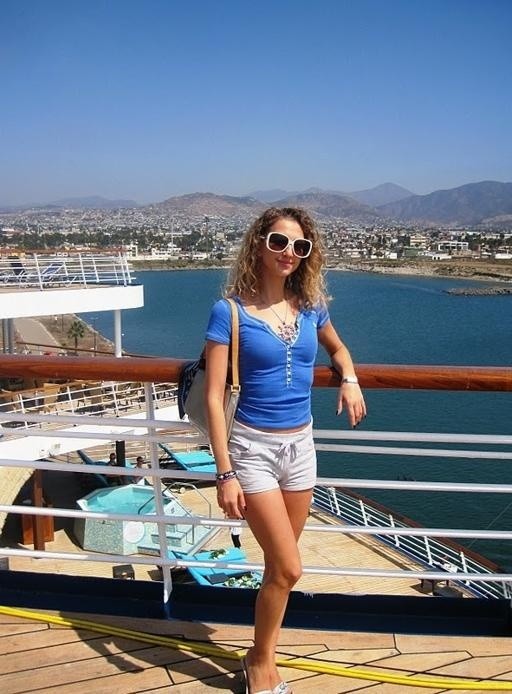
[148,443,218,489]
[77,450,134,485]
[168,546,263,589]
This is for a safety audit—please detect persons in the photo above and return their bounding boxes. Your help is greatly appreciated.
[134,455,145,482]
[106,453,123,484]
[202,204,369,694]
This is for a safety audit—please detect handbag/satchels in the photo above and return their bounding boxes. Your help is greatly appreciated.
[184,297,241,446]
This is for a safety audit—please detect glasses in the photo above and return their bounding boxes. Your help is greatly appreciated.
[260,232,312,258]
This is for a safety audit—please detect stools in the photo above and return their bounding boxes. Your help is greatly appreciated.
[433,586,463,598]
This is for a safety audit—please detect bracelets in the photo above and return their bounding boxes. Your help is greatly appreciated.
[340,376,359,385]
[213,468,238,482]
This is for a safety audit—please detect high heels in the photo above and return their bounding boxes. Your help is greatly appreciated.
[240,652,294,694]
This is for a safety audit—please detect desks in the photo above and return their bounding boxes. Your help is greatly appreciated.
[420,568,450,591]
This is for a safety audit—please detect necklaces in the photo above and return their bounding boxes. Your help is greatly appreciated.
[261,289,296,339]
